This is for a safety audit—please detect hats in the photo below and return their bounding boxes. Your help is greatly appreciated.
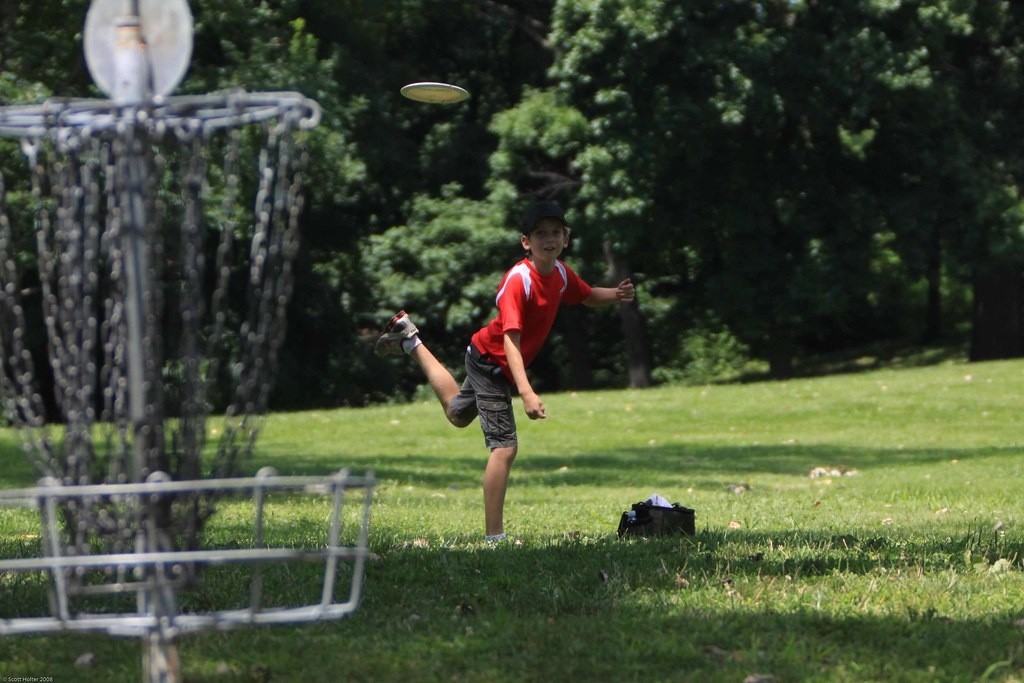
[522,200,564,236]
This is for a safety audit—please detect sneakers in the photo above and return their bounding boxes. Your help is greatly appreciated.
[374,309,420,358]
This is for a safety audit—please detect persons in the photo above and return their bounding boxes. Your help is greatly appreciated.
[373,198,635,543]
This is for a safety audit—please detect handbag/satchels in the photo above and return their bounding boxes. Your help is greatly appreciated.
[616,497,695,536]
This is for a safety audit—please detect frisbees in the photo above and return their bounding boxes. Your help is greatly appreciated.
[400,81,470,104]
